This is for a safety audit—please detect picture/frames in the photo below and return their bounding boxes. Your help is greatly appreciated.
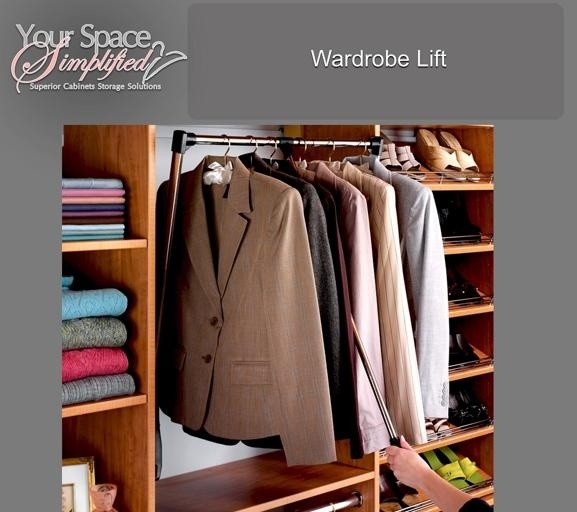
[61,454,96,512]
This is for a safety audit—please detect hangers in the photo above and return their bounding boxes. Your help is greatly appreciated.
[201,134,368,172]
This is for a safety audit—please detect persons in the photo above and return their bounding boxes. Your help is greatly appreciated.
[385,434,493,512]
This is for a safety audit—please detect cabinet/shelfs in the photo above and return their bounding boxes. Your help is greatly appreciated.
[61,123,156,511]
[281,123,494,512]
[155,451,374,511]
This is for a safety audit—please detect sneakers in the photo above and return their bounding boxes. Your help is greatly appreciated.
[425,417,452,442]
[448,384,491,430]
[447,274,484,306]
[437,203,481,245]
[449,328,480,371]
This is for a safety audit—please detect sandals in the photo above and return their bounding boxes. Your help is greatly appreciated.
[380,131,409,178]
[395,142,427,182]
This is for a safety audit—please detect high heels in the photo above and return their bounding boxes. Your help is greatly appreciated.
[438,131,481,184]
[414,127,467,182]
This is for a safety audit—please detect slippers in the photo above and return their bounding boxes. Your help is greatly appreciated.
[379,474,403,512]
[438,446,486,488]
[386,467,423,510]
[422,450,470,490]
